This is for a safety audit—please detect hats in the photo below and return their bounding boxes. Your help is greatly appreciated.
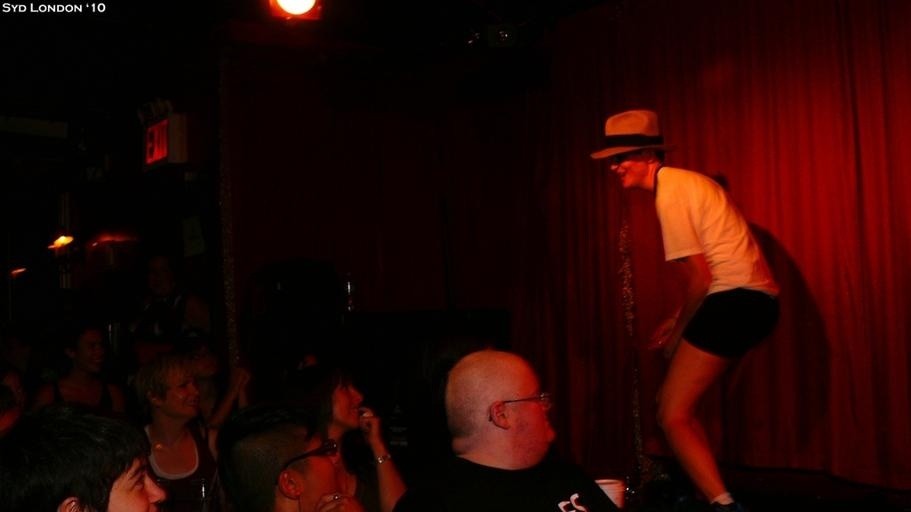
[591,110,679,160]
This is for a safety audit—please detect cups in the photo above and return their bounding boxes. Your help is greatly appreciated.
[594,480,624,511]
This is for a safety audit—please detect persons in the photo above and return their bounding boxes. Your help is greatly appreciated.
[0,248,407,512]
[591,110,781,512]
[391,349,620,512]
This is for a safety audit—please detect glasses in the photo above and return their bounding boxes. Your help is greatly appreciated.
[489,393,553,422]
[273,440,338,485]
[615,151,641,163]
